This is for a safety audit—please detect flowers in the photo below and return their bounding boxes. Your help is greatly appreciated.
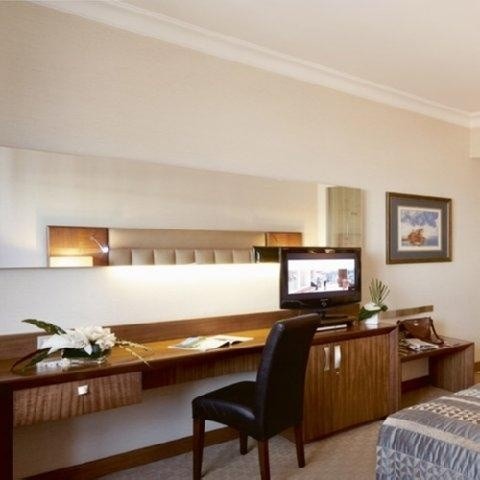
[10,318,151,369]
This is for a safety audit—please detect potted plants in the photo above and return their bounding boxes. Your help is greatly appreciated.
[358,277,390,324]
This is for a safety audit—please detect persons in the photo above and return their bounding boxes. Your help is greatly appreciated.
[321,272,328,291]
[310,270,321,291]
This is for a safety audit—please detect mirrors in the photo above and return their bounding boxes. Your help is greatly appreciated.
[0,145,365,269]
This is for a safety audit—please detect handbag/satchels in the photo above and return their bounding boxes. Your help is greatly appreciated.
[395,316,444,345]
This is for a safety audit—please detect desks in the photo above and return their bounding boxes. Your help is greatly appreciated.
[1,316,401,480]
[400,336,475,392]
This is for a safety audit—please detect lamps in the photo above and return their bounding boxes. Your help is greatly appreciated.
[90,235,108,254]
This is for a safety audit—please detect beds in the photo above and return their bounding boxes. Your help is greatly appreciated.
[374,382,480,479]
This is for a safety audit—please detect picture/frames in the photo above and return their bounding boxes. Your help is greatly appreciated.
[384,192,452,263]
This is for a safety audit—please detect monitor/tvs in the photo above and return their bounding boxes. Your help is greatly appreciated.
[278,245,363,328]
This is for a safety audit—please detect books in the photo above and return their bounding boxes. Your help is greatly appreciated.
[167,333,255,352]
[402,337,439,352]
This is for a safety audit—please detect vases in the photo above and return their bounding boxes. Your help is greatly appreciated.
[60,346,112,366]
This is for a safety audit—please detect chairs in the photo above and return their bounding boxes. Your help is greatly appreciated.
[191,313,321,480]
